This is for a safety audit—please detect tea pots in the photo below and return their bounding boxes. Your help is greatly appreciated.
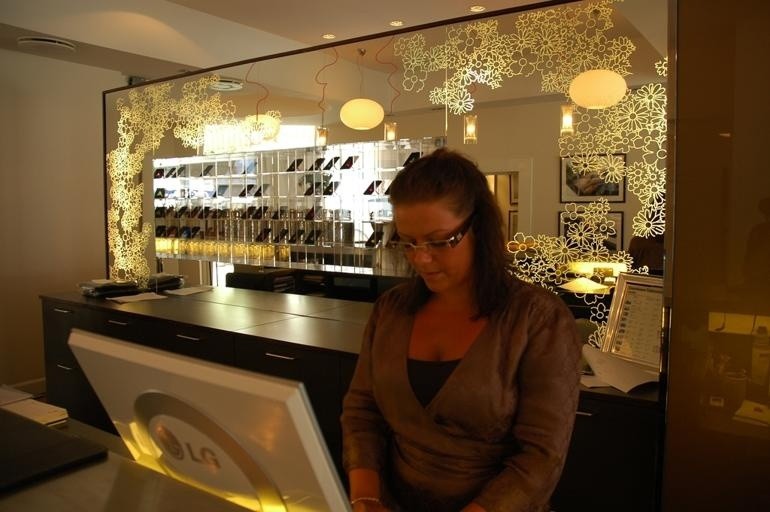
[102,0,672,352]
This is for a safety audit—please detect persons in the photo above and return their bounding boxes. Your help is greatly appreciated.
[338,145,584,512]
[623,197,666,274]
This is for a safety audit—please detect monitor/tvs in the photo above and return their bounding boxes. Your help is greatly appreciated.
[0,398,70,427]
[0,384,35,406]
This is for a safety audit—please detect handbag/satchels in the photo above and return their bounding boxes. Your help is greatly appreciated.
[348,494,384,504]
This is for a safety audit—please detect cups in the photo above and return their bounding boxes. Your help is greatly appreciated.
[388,213,475,262]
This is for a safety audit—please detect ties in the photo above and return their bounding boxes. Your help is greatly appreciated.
[66,328,353,512]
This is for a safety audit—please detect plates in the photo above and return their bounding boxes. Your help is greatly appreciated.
[37,291,663,511]
[152,135,447,279]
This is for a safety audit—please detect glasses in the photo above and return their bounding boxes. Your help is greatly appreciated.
[462,22,480,145]
[315,35,398,146]
[558,7,629,136]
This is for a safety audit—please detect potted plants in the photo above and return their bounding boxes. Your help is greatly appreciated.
[600,271,663,374]
[557,154,628,251]
